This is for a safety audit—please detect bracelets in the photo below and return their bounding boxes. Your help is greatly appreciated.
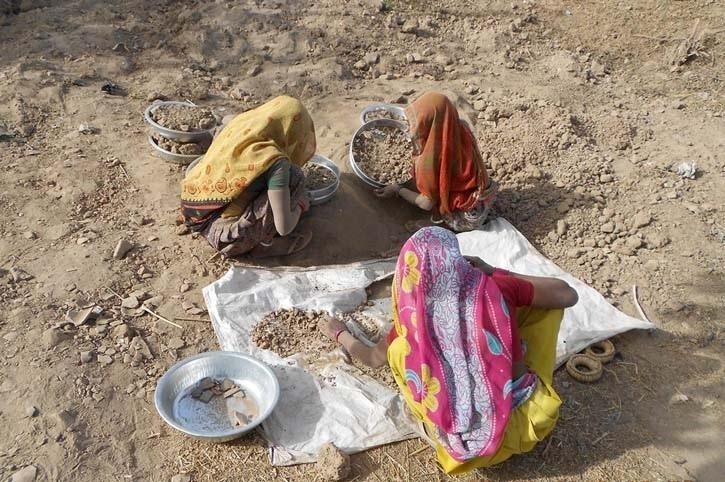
[395,186,404,198]
[335,329,346,341]
[296,200,306,212]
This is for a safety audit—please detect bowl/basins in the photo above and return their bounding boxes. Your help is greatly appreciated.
[360,104,405,124]
[153,351,280,444]
[310,182,341,210]
[146,102,216,140]
[300,152,340,198]
[349,119,420,190]
[148,132,202,164]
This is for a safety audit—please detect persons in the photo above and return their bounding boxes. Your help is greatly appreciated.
[317,226,579,476]
[180,95,317,261]
[375,92,502,233]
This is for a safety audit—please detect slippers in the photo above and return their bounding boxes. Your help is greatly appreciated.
[282,229,313,256]
[401,400,433,446]
[404,218,427,233]
